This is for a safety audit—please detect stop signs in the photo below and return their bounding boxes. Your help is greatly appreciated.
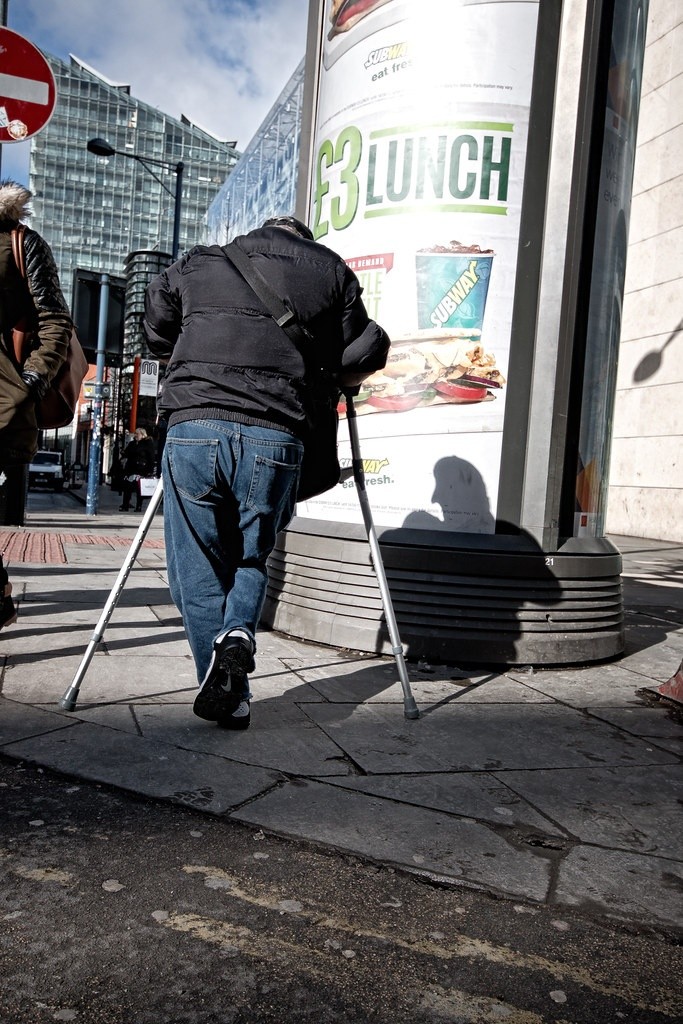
[0,25,58,144]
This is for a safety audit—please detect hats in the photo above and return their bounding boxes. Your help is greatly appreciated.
[262,215,315,242]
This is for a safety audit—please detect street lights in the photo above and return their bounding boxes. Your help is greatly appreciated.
[89,127,189,273]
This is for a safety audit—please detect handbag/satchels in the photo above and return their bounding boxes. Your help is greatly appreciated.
[11,221,89,431]
[293,374,342,504]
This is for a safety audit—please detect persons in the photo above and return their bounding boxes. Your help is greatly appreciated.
[140,216,391,730]
[0,183,73,631]
[120,428,153,512]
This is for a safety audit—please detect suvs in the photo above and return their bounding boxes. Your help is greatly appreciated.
[27,451,66,493]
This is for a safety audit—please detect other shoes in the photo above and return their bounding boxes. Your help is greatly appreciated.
[0,582,18,633]
[119,505,128,511]
[134,506,141,512]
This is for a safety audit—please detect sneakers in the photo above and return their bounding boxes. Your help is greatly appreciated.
[221,700,255,732]
[192,627,253,721]
